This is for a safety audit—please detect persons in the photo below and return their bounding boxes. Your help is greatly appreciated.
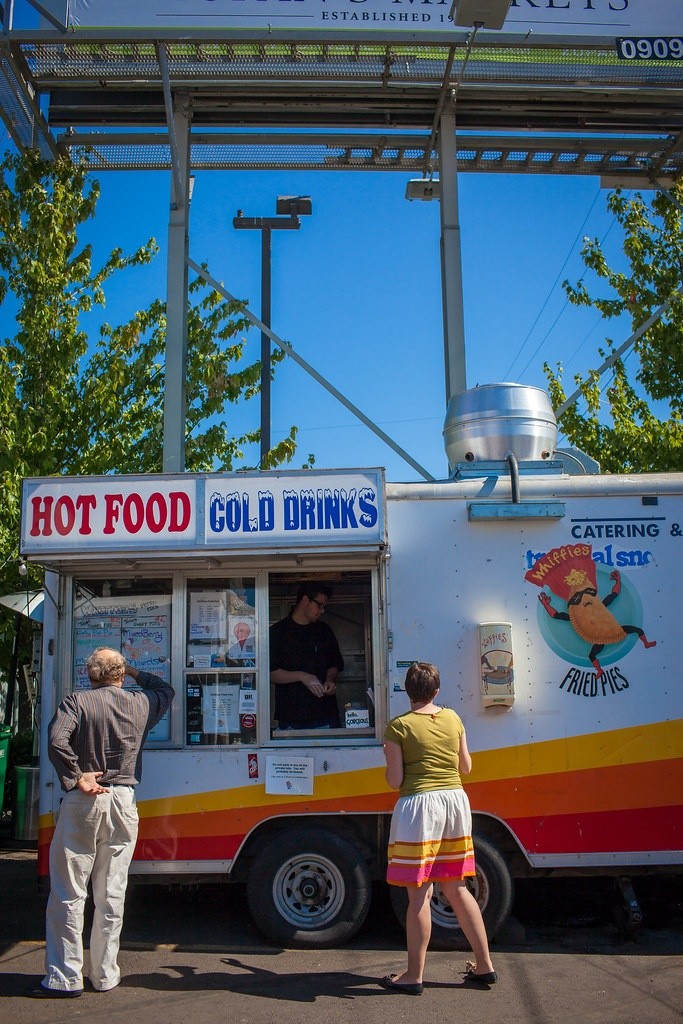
[269,580,344,731]
[24,647,176,998]
[379,661,498,995]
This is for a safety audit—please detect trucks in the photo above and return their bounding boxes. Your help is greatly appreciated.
[26,458,681,950]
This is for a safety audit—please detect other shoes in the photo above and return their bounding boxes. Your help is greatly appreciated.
[463,968,498,984]
[378,973,424,997]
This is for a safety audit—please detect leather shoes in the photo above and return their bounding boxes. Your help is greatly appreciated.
[23,980,82,997]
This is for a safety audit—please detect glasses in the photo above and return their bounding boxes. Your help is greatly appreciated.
[310,598,327,610]
